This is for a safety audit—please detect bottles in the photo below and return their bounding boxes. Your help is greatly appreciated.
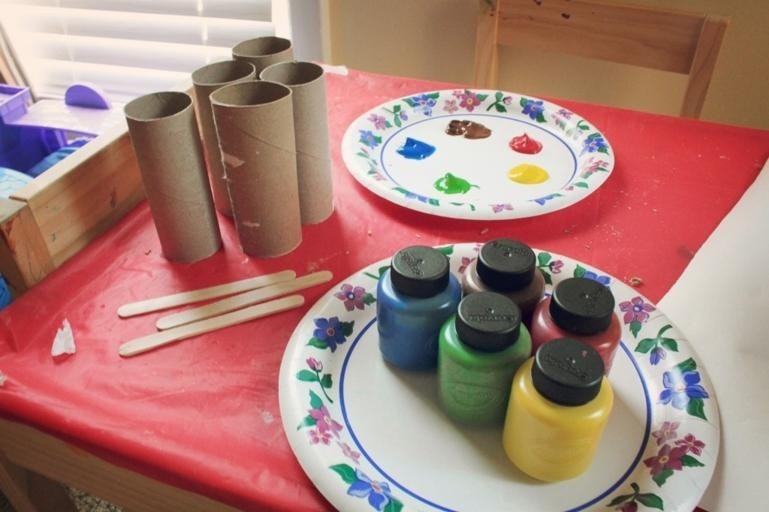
[503,339,615,481]
[376,245,460,374]
[438,291,533,427]
[461,241,546,328]
[529,277,621,379]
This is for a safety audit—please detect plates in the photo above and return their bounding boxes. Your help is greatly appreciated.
[279,243,722,512]
[339,87,616,221]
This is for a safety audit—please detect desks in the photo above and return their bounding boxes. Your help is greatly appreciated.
[4,63,768,512]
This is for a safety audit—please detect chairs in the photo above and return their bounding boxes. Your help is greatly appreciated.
[472,0,729,119]
